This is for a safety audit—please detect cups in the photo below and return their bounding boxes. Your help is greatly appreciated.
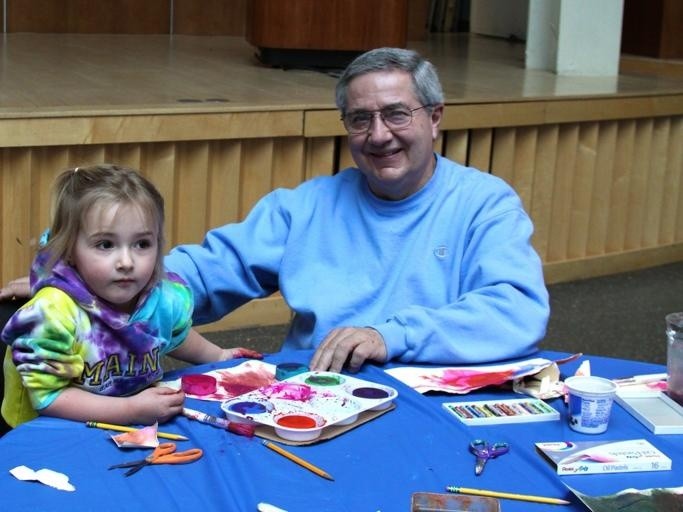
[563,374,619,435]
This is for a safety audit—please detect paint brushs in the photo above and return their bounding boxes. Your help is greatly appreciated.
[181,407,256,438]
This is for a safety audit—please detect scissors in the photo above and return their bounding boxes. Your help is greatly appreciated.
[469,439,509,475]
[108,443,203,477]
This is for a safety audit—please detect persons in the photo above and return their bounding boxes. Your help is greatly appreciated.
[0,162,266,435]
[152,46,552,376]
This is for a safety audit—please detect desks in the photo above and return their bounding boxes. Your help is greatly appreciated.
[1,346,683,512]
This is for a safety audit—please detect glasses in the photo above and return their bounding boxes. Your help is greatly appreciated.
[341,103,431,135]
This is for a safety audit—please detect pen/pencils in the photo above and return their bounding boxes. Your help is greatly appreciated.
[86,421,189,441]
[446,486,571,505]
[262,440,335,481]
[448,400,554,418]
